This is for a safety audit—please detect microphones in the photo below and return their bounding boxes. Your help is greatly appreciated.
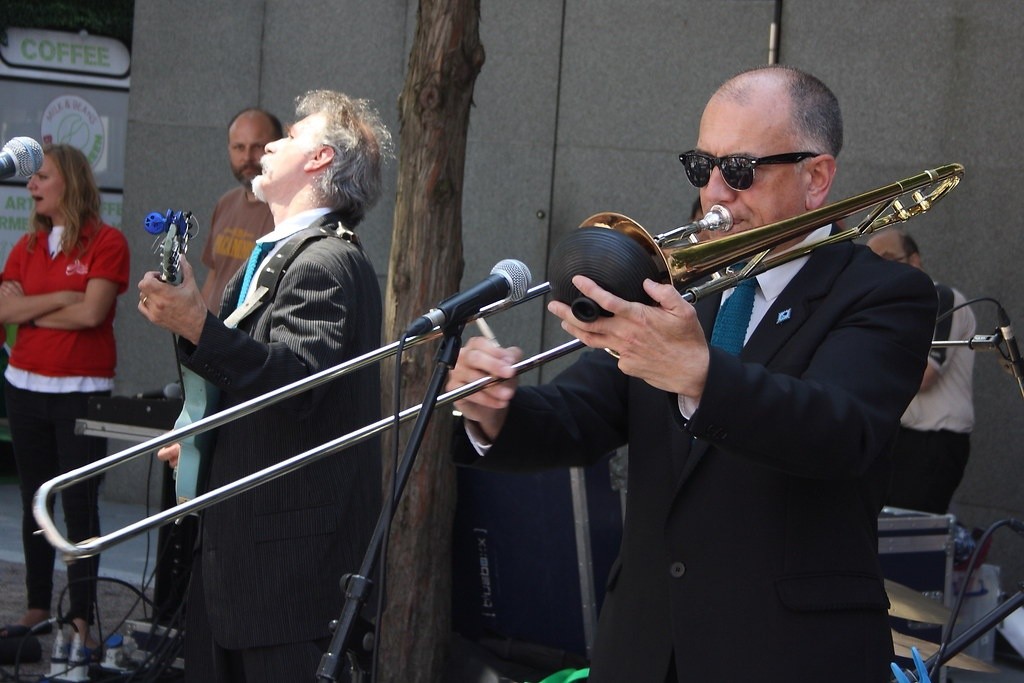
[405,259,532,336]
[999,305,1024,397]
[0,136,44,179]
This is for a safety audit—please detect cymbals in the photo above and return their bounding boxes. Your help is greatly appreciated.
[881,578,962,625]
[889,627,1001,674]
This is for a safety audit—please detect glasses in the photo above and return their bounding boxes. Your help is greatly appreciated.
[677,148,821,192]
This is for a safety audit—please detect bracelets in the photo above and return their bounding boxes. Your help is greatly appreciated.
[28,319,38,328]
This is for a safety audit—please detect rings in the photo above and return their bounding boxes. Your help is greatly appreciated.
[143,296,148,304]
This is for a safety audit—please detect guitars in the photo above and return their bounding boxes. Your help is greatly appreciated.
[141,208,220,518]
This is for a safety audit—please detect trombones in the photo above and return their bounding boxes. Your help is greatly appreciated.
[29,160,965,564]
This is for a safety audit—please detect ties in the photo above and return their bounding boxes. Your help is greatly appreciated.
[712,263,763,361]
[232,240,277,330]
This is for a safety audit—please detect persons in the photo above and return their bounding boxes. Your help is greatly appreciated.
[197,106,285,320]
[867,226,980,597]
[136,86,396,683]
[690,197,710,240]
[442,57,936,683]
[0,145,134,657]
